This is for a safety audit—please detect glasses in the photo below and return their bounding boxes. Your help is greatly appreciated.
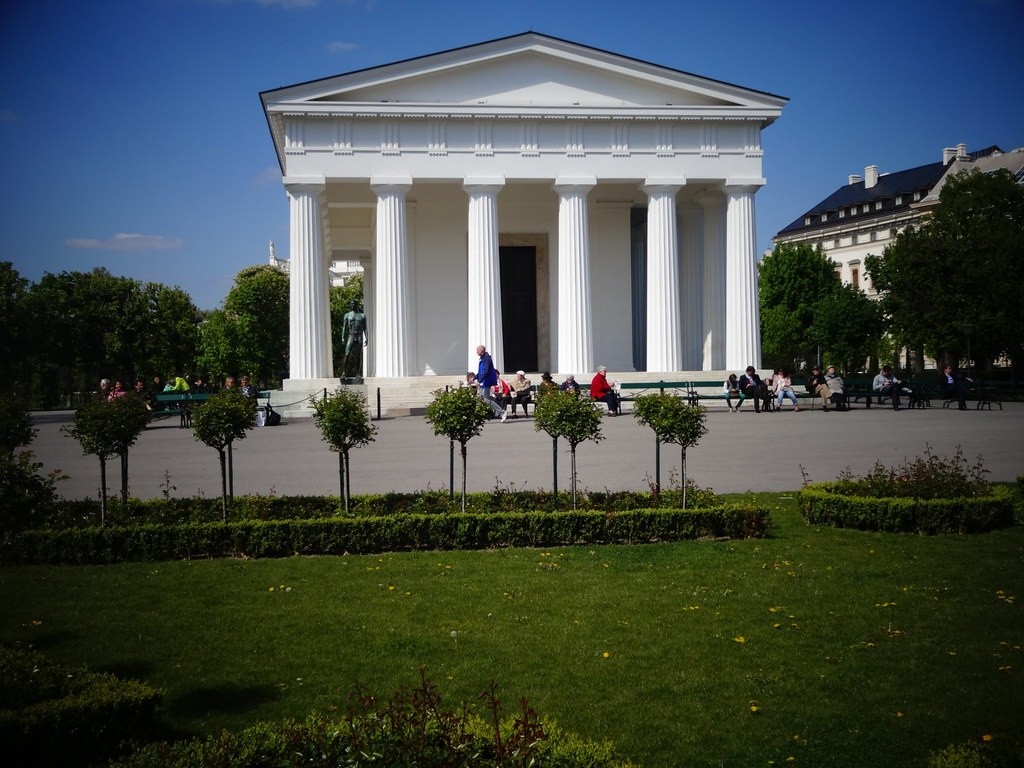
[812,369,818,371]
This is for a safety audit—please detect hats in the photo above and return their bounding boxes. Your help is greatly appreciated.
[517,370,526,376]
[541,372,552,379]
[826,365,834,370]
[812,365,818,369]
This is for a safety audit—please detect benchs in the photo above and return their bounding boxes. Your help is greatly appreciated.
[152,393,270,428]
[506,378,1002,414]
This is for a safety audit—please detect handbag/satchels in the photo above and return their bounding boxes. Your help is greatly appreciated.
[731,391,738,397]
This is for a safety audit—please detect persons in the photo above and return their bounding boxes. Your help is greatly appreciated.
[872,366,911,411]
[723,366,799,413]
[805,364,845,412]
[97,373,257,407]
[340,298,368,380]
[467,346,618,423]
[940,365,974,410]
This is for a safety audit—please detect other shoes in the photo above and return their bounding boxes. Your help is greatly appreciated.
[830,397,839,404]
[607,412,618,417]
[824,406,829,412]
[894,406,899,411]
[959,406,968,411]
[501,411,508,423]
[755,409,760,413]
[898,402,902,405]
[795,406,799,412]
[836,405,848,411]
[511,415,518,419]
[526,414,529,418]
[729,407,733,413]
[735,407,740,413]
